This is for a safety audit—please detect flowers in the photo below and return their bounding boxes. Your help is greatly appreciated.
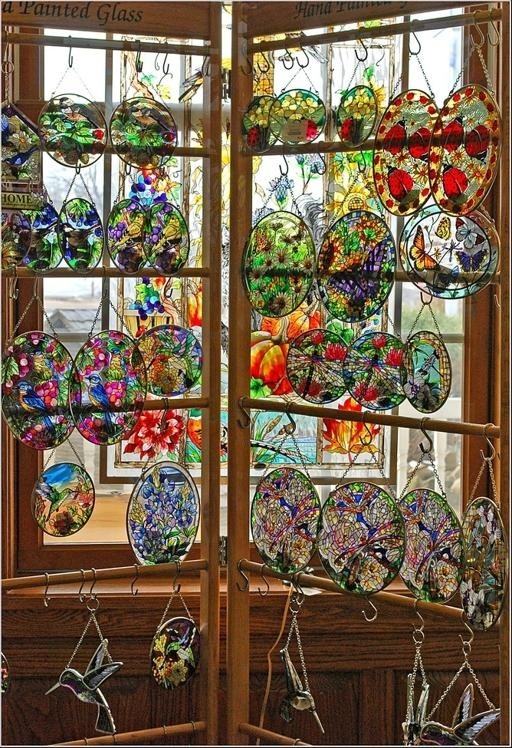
[252,415,313,464]
[244,220,314,313]
[154,636,195,687]
[320,395,380,463]
[327,72,385,225]
[240,101,277,150]
[40,98,103,165]
[342,89,372,146]
[111,98,175,165]
[318,216,392,317]
[403,336,444,408]
[2,211,31,268]
[6,124,38,180]
[272,91,318,141]
[348,337,405,404]
[293,331,344,401]
[123,409,186,462]
